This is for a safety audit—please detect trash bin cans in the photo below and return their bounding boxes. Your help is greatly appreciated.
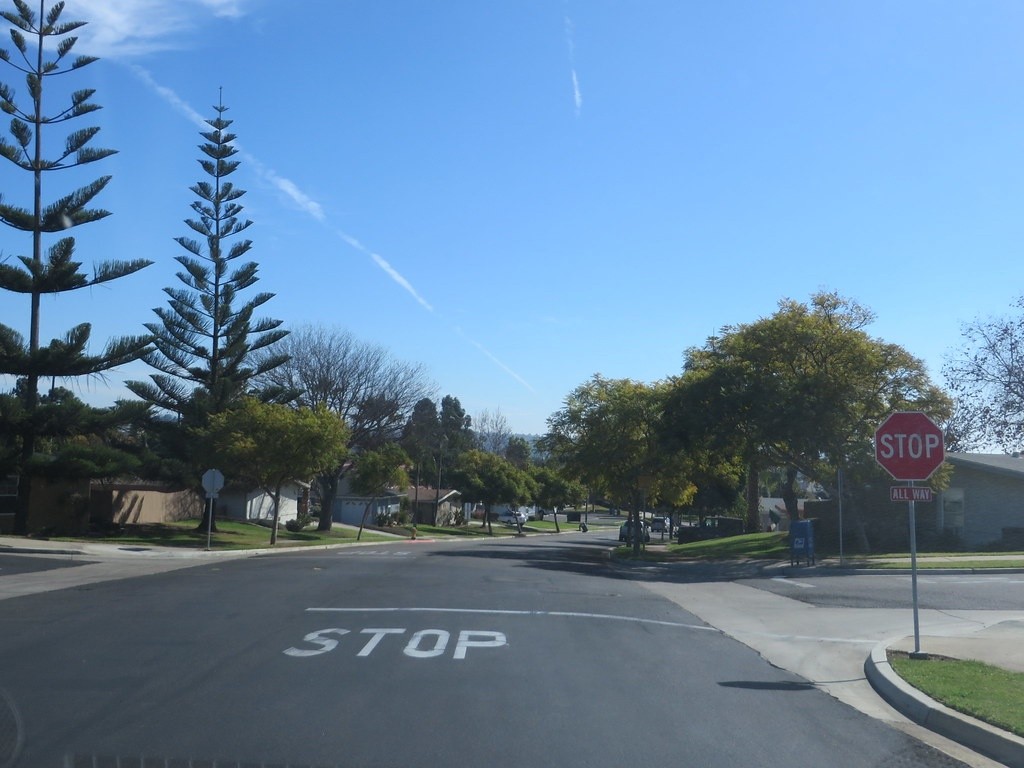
[790,520,815,558]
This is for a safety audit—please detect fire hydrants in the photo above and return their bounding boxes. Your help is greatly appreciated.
[410,526,418,540]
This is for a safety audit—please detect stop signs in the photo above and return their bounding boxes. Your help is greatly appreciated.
[874,411,945,482]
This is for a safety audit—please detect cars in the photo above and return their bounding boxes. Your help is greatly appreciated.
[496,510,526,525]
[619,520,651,542]
[650,517,675,533]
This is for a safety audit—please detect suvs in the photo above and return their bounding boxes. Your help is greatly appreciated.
[673,515,745,545]
